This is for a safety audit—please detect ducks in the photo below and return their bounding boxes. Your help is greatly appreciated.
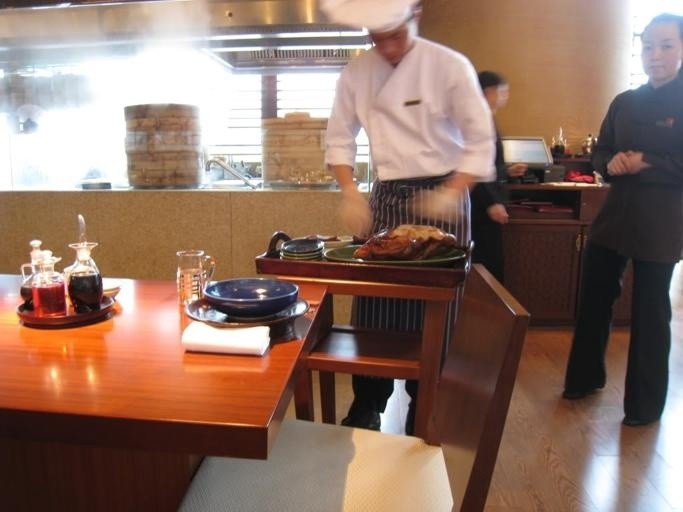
[351,223,458,261]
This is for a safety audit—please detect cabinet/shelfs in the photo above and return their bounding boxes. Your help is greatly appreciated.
[500,185,633,326]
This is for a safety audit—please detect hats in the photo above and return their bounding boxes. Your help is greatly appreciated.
[478,69,508,90]
[320,0,419,37]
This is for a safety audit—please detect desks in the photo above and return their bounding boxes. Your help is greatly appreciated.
[250,254,457,438]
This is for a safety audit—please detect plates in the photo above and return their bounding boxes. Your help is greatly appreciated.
[16,296,115,327]
[186,298,309,327]
[279,236,467,266]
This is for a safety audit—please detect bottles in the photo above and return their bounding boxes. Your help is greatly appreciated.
[20,240,102,317]
[551,132,598,156]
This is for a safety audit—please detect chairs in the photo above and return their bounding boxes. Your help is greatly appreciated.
[171,260,533,512]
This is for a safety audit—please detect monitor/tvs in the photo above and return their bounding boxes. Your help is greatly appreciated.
[500,136,553,170]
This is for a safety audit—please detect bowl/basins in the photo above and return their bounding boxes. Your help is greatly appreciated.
[203,278,298,320]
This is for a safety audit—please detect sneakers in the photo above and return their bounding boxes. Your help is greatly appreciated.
[560,377,607,399]
[619,413,663,425]
[339,405,382,431]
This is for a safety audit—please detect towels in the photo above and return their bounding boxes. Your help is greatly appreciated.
[182,319,269,357]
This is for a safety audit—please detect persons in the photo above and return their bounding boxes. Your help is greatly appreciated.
[560,10,683,428]
[321,0,496,444]
[466,70,530,283]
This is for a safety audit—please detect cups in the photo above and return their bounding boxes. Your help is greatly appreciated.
[175,249,215,305]
